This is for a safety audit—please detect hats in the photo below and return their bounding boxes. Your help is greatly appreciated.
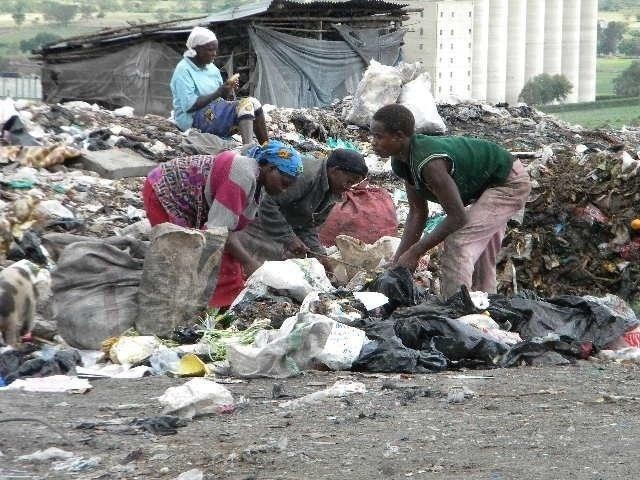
[329,149,369,173]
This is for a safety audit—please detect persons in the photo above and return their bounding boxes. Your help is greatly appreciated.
[253,148,369,282]
[170,25,268,148]
[367,103,534,306]
[143,137,303,317]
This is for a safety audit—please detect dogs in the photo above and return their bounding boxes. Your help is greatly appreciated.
[0,258,40,349]
